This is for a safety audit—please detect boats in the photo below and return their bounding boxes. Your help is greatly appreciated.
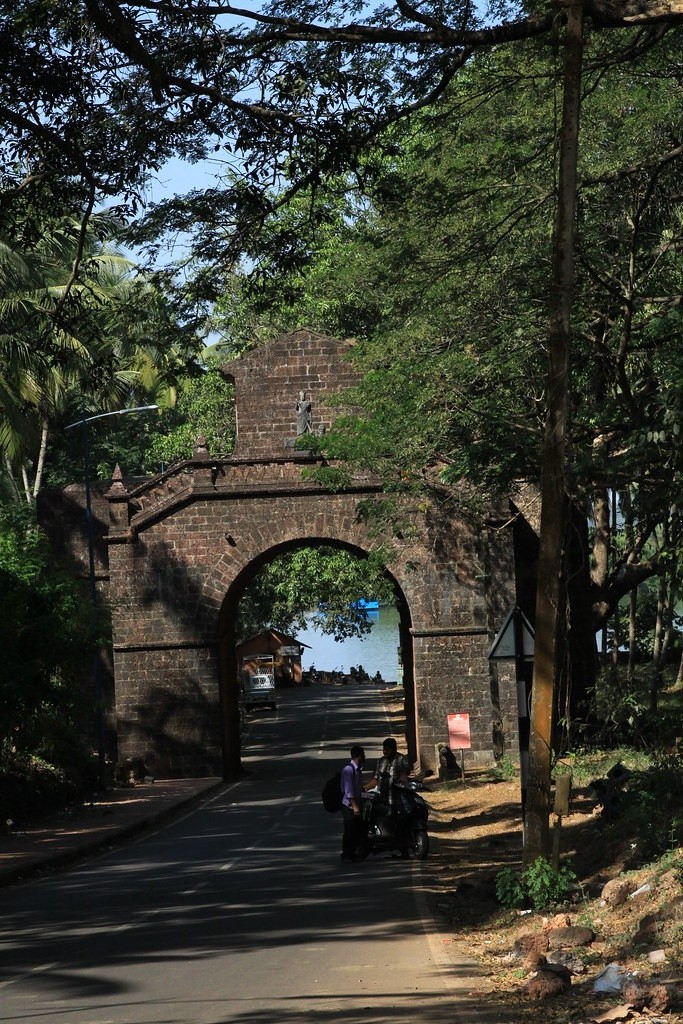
[318,600,379,612]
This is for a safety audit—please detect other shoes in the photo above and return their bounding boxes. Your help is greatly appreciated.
[341,852,360,862]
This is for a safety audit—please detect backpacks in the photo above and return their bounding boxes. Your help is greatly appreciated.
[321,763,355,814]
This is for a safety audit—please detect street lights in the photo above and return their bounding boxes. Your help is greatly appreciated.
[83,404,159,798]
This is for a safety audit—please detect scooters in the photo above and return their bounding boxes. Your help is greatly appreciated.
[354,769,434,860]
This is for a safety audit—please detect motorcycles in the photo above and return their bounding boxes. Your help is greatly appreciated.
[243,653,276,712]
[305,663,322,682]
[331,665,363,685]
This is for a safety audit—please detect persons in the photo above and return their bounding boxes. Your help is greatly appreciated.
[362,738,411,860]
[350,665,381,681]
[339,745,366,863]
